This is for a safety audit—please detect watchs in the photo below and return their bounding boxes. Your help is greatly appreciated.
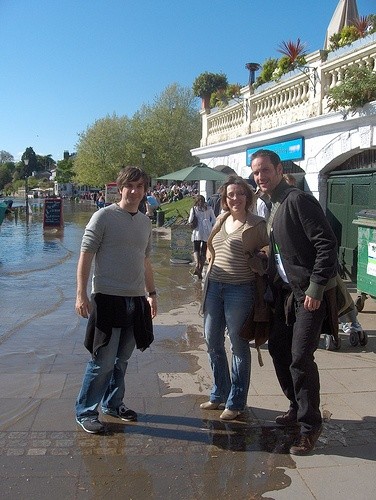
[146,291,157,297]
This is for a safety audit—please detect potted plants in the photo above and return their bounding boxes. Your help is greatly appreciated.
[191,71,228,109]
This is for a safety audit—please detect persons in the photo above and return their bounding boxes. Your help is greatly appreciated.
[187,173,298,279]
[199,176,272,420]
[250,149,358,456]
[75,167,157,433]
[79,181,198,209]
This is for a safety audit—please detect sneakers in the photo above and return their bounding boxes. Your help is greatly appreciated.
[74,410,105,434]
[103,402,138,424]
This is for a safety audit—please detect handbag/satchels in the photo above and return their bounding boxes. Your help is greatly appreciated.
[333,277,353,321]
[238,301,273,345]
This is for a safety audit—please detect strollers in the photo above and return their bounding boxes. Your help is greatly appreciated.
[324,317,368,351]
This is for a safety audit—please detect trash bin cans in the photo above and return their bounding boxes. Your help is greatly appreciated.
[351,208,376,312]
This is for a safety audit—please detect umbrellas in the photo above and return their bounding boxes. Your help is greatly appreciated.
[153,163,227,196]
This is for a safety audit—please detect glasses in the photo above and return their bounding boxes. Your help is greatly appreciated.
[226,192,248,199]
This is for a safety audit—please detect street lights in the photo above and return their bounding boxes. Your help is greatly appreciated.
[140,150,146,171]
[22,154,30,216]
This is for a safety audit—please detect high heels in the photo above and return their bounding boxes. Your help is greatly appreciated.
[200,395,244,421]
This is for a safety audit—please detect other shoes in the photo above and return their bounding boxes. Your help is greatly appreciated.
[275,408,300,424]
[290,422,323,455]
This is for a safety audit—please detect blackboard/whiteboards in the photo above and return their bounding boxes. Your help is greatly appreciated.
[44,199,61,227]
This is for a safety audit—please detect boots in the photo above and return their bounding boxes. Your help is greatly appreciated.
[192,251,206,279]
